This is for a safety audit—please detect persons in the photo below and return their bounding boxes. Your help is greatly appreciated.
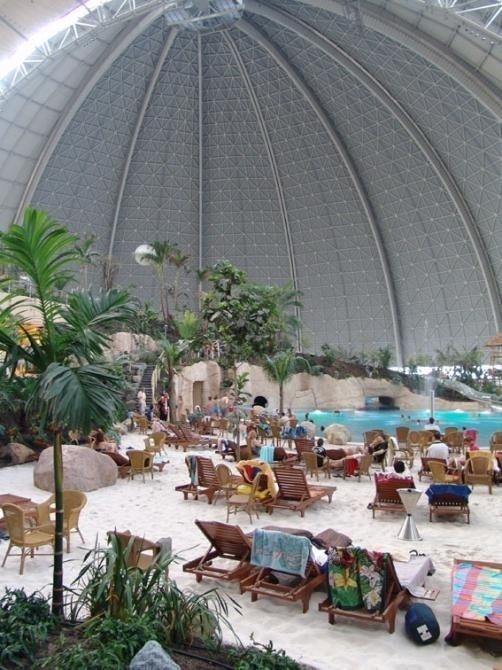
[313,439,327,468]
[389,409,501,492]
[126,385,300,456]
[320,427,326,431]
[351,433,388,463]
[322,446,372,469]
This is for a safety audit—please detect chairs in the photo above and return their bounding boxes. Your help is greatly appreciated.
[316,544,433,635]
[104,528,173,583]
[238,527,354,614]
[2,489,89,576]
[443,555,501,646]
[181,517,313,584]
[67,414,501,524]
